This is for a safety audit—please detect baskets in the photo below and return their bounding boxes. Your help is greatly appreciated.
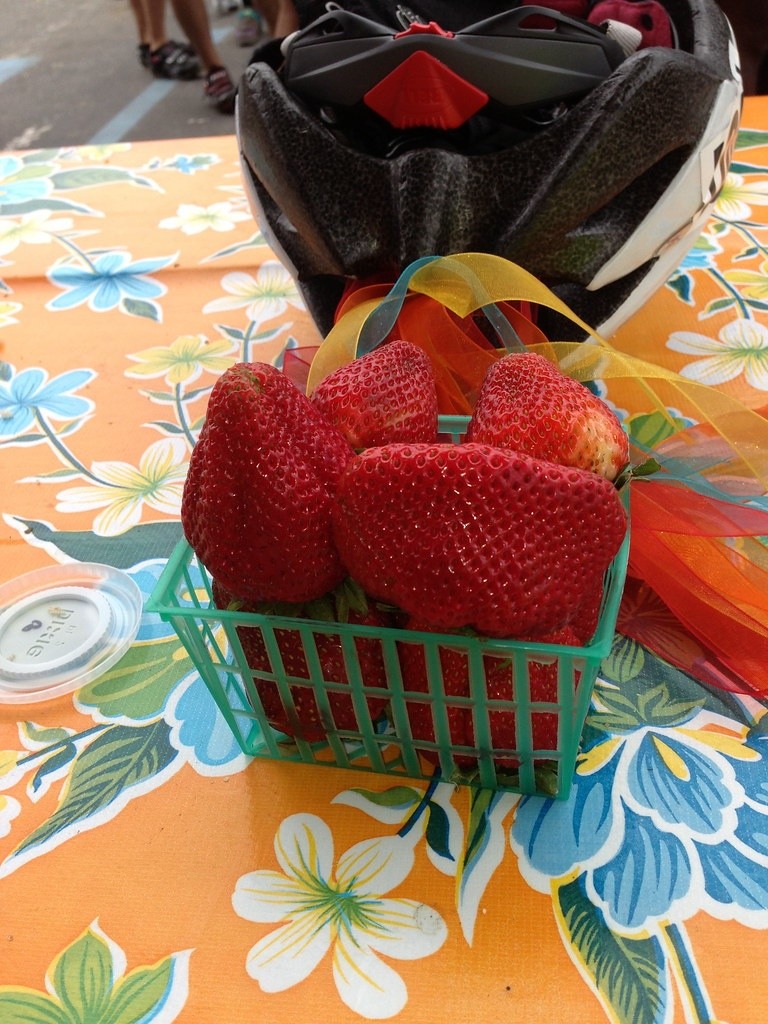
[148,413,633,801]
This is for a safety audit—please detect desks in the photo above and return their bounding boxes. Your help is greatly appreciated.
[1,93,768,1024]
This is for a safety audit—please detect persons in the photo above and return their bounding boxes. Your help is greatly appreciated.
[170,1,296,109]
[129,0,207,81]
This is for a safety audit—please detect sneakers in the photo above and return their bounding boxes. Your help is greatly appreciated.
[234,7,262,46]
[204,64,236,115]
[139,36,200,80]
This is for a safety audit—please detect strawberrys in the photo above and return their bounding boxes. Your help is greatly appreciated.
[178,340,629,767]
